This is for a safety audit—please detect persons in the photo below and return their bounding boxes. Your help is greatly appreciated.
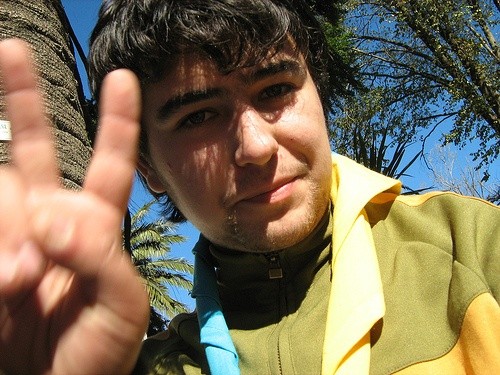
[2,0,500,375]
